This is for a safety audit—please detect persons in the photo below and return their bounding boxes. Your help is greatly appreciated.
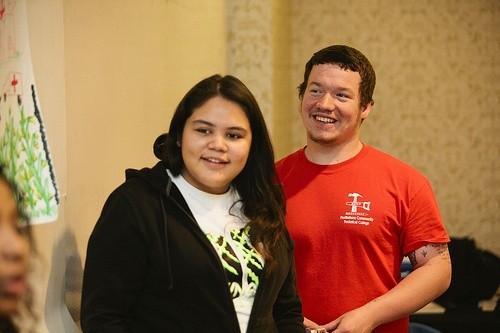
[275,45,453,333]
[80,73,306,333]
[0,164,43,333]
